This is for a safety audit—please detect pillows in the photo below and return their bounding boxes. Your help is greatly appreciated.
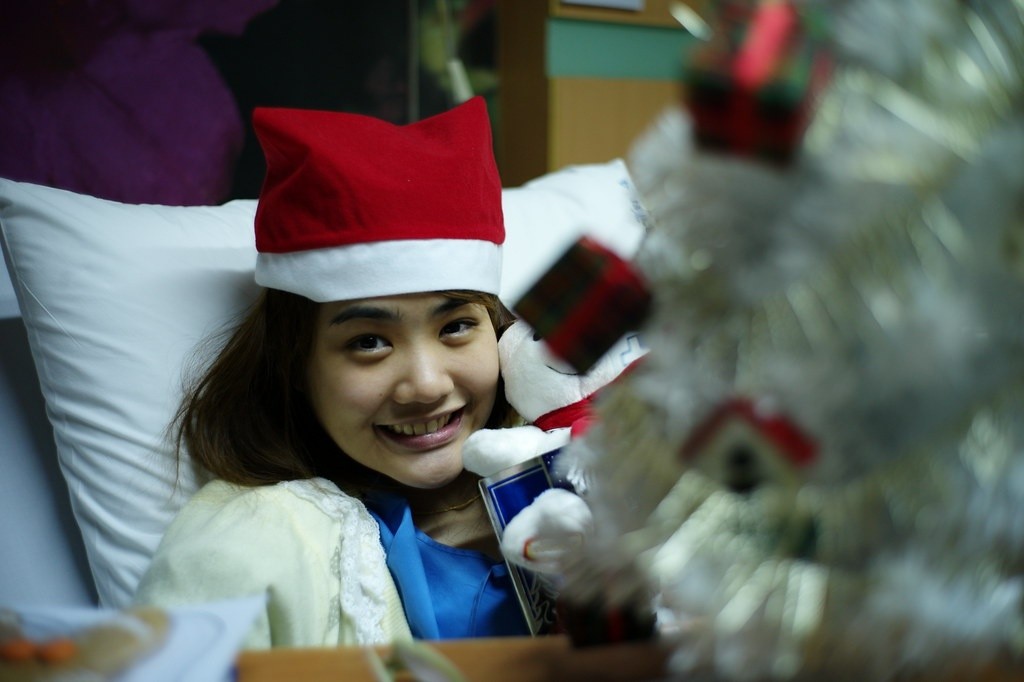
[0,156,662,613]
[0,316,100,618]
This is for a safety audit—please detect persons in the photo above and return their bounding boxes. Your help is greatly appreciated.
[129,94,532,649]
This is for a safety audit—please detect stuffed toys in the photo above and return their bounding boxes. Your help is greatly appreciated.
[467,312,650,579]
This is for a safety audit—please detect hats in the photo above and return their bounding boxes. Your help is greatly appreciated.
[252,95,506,303]
